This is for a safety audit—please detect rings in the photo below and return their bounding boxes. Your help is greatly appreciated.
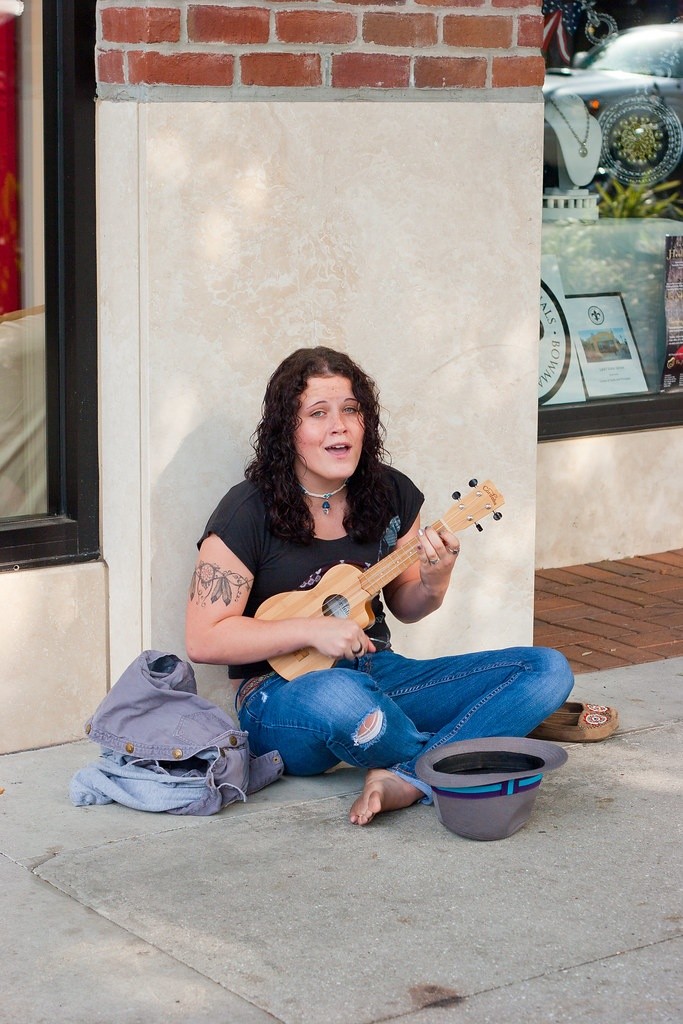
[353,643,363,654]
[449,548,460,554]
[429,558,438,564]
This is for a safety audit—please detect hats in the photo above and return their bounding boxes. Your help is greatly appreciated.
[415,735,568,841]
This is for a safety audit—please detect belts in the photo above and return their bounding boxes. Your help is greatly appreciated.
[236,669,277,715]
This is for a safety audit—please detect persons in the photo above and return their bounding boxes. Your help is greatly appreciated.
[185,346,574,825]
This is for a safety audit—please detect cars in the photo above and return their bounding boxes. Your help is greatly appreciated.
[543,24,683,186]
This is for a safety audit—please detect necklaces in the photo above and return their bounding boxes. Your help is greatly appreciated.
[547,94,589,157]
[298,479,348,515]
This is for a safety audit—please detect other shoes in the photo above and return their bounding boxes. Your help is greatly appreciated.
[525,711,620,742]
[555,701,618,718]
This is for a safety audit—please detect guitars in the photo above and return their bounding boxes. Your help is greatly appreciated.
[252,477,506,681]
